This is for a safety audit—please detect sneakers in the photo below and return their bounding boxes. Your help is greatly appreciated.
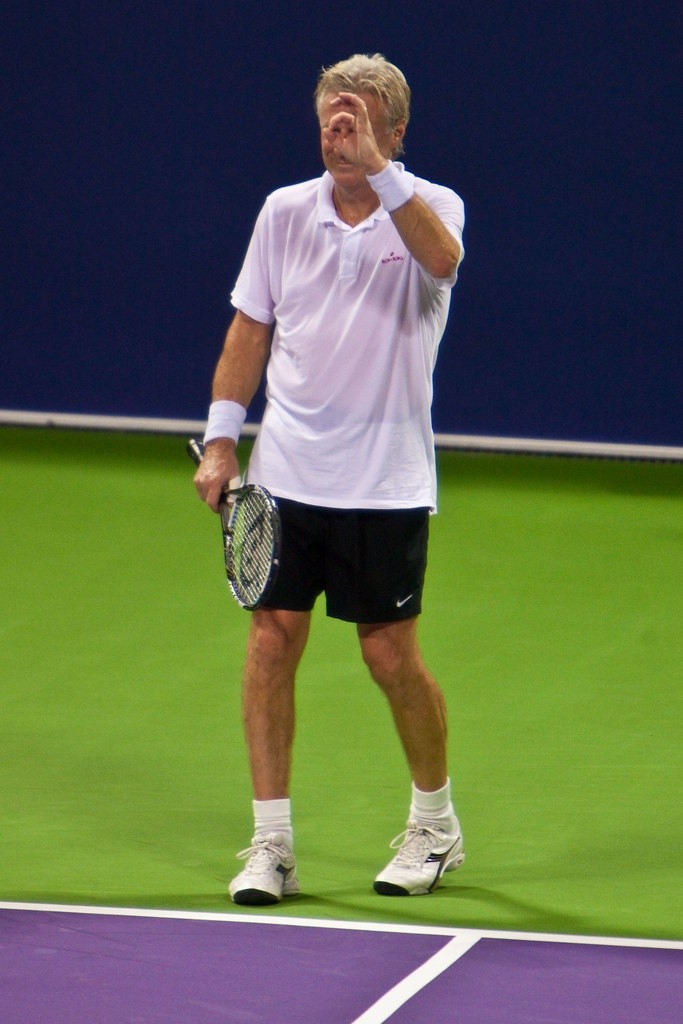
[371,818,466,898]
[229,832,301,904]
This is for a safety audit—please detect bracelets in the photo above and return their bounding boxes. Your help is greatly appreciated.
[203,401,247,446]
[365,160,412,212]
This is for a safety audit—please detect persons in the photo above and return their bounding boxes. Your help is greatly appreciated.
[193,48,465,906]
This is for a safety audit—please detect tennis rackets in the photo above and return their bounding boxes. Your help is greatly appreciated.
[186,436,282,613]
